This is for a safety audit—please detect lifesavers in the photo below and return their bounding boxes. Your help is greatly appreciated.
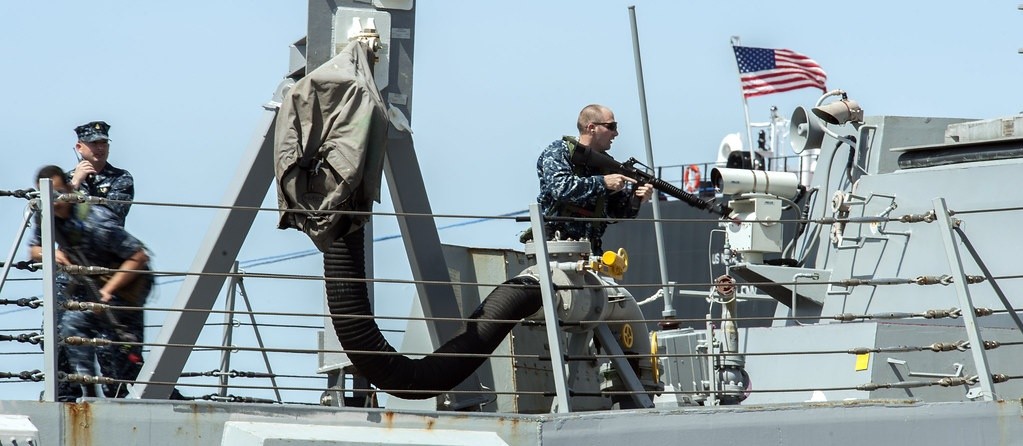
[684,164,701,193]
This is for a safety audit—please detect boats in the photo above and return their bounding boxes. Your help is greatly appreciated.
[0,4,1023,445]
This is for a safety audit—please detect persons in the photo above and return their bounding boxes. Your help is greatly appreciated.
[537,105,653,355]
[28,121,154,402]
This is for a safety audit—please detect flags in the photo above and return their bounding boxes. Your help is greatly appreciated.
[733,45,827,98]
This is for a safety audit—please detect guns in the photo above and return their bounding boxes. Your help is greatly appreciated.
[567,141,745,228]
[56,231,146,368]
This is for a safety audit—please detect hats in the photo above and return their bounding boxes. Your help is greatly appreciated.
[73,121,113,143]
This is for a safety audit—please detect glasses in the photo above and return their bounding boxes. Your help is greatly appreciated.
[586,120,618,132]
